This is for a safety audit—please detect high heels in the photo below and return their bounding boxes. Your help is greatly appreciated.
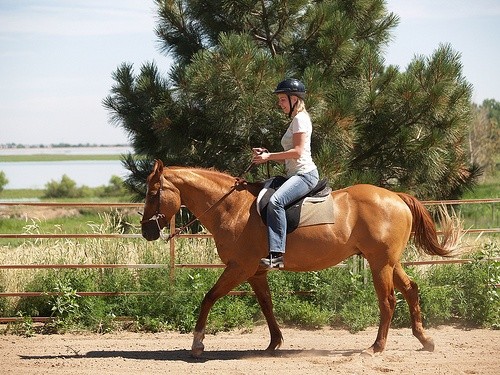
[260,252,284,269]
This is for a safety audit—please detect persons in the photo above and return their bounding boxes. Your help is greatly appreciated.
[251,77,320,270]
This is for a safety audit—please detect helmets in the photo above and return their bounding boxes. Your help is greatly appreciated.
[274,78,305,97]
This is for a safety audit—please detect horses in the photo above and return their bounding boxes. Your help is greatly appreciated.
[141,157,485,360]
